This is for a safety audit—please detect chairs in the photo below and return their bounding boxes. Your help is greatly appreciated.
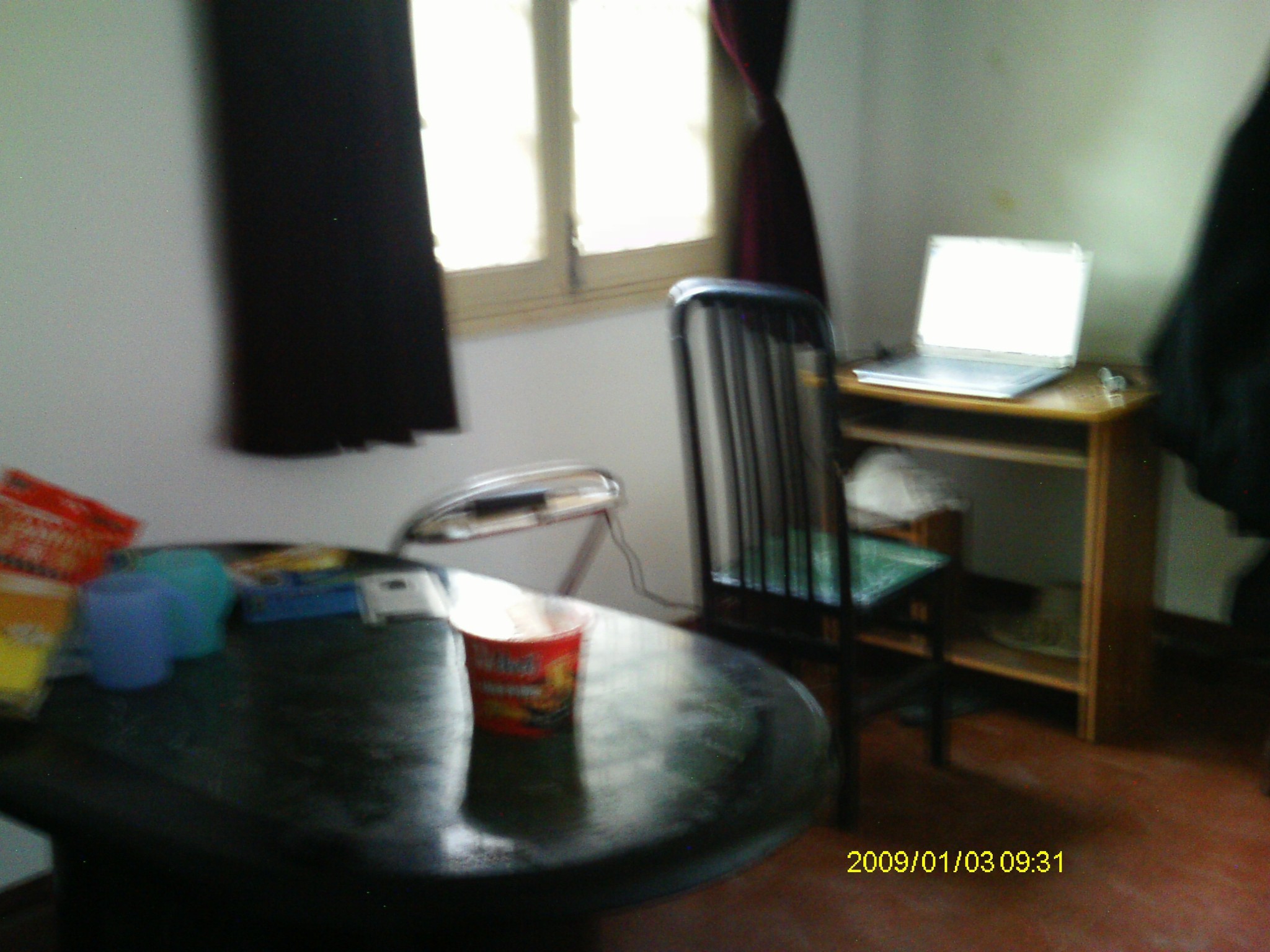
[668,275,944,835]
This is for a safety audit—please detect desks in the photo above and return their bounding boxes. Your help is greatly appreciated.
[788,349,1170,744]
[0,543,829,952]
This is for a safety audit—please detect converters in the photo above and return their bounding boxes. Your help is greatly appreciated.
[472,487,548,518]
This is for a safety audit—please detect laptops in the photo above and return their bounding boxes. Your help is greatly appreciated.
[850,235,1090,397]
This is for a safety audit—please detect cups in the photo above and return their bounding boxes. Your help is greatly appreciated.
[135,550,234,659]
[80,574,201,687]
